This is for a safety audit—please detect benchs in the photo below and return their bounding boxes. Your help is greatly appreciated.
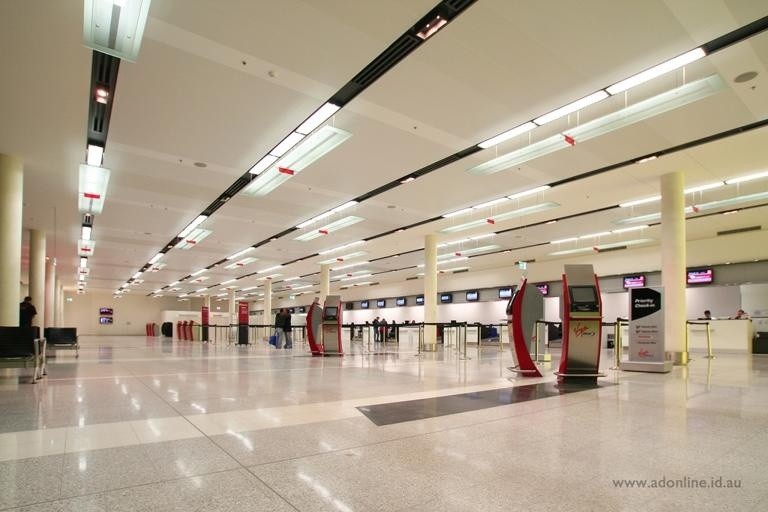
[0,325,47,384]
[44,328,80,358]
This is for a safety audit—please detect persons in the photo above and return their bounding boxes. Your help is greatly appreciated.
[734,309,744,319]
[703,310,717,320]
[274,309,285,349]
[351,322,356,341]
[372,317,382,342]
[20,296,37,328]
[366,321,371,327]
[388,320,395,338]
[380,318,387,325]
[281,309,292,349]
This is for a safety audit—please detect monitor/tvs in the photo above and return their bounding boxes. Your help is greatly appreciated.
[466,291,479,300]
[416,297,424,303]
[538,284,549,294]
[685,270,713,285]
[100,308,113,314]
[568,285,598,311]
[345,302,353,309]
[396,298,406,306]
[441,294,452,302]
[289,306,305,314]
[377,300,385,307]
[361,301,368,308]
[325,306,338,319]
[623,275,645,288]
[497,287,513,298]
[100,316,113,324]
[506,290,518,312]
